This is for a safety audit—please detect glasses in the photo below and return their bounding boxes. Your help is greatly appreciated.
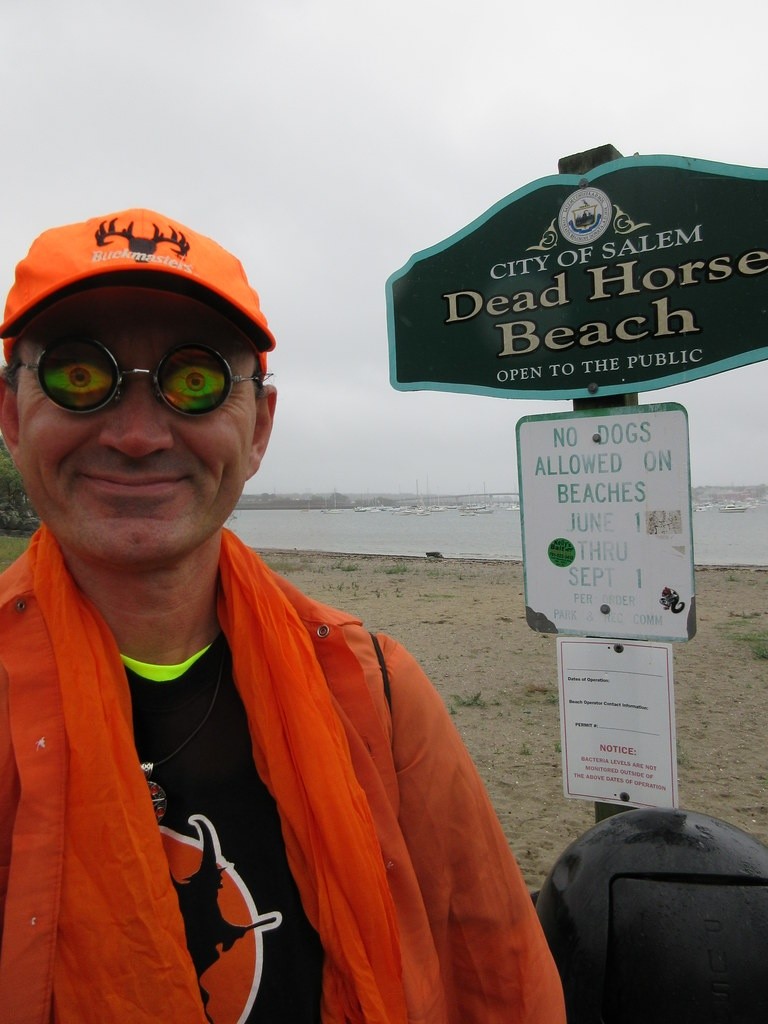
[13,337,272,415]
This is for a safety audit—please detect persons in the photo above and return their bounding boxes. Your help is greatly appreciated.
[1,210,572,1024]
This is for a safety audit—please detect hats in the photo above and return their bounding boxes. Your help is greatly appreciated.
[1,208,275,379]
